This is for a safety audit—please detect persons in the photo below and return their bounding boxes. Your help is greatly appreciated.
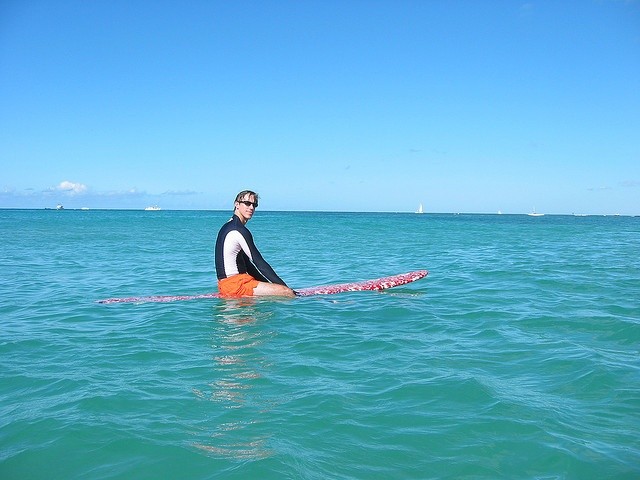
[214,189,299,299]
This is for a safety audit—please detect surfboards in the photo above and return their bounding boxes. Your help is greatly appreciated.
[96,270,429,305]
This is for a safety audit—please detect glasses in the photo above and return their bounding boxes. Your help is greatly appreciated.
[238,201,257,207]
